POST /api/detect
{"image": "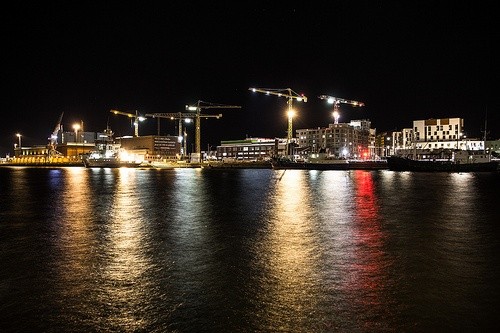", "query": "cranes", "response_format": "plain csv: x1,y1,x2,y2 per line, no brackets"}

49,112,65,150
109,109,147,137
185,100,242,153
248,87,308,141
145,111,219,152
319,95,365,124
